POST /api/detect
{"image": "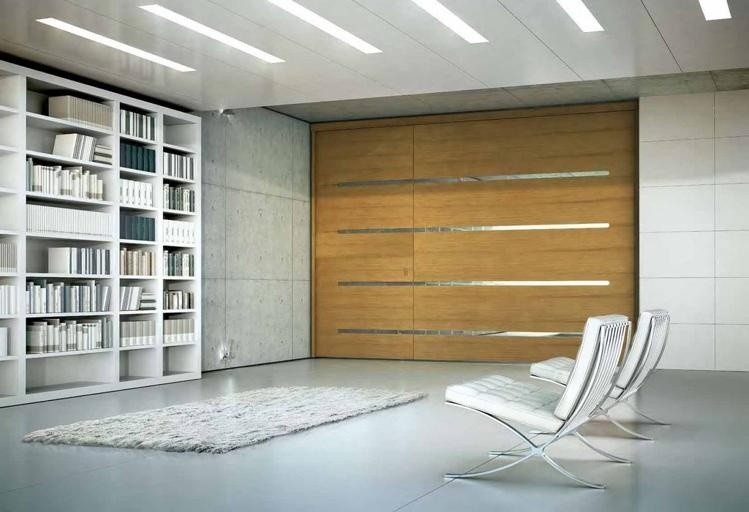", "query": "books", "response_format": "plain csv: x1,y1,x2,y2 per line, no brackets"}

1,82,198,355
